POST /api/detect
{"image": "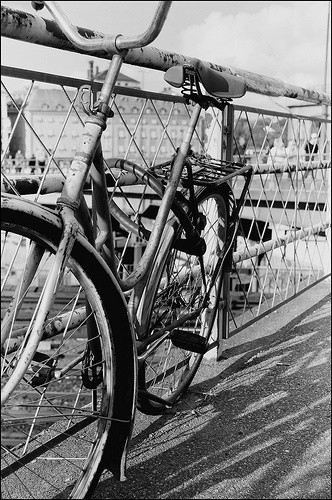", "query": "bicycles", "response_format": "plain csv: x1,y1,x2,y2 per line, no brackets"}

0,1,253,500
